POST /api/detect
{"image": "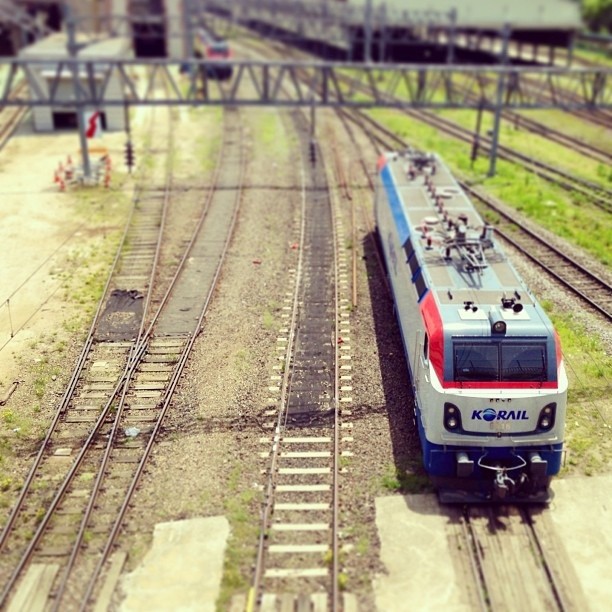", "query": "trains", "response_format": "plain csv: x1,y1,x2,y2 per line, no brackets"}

192,25,233,78
375,150,568,503
